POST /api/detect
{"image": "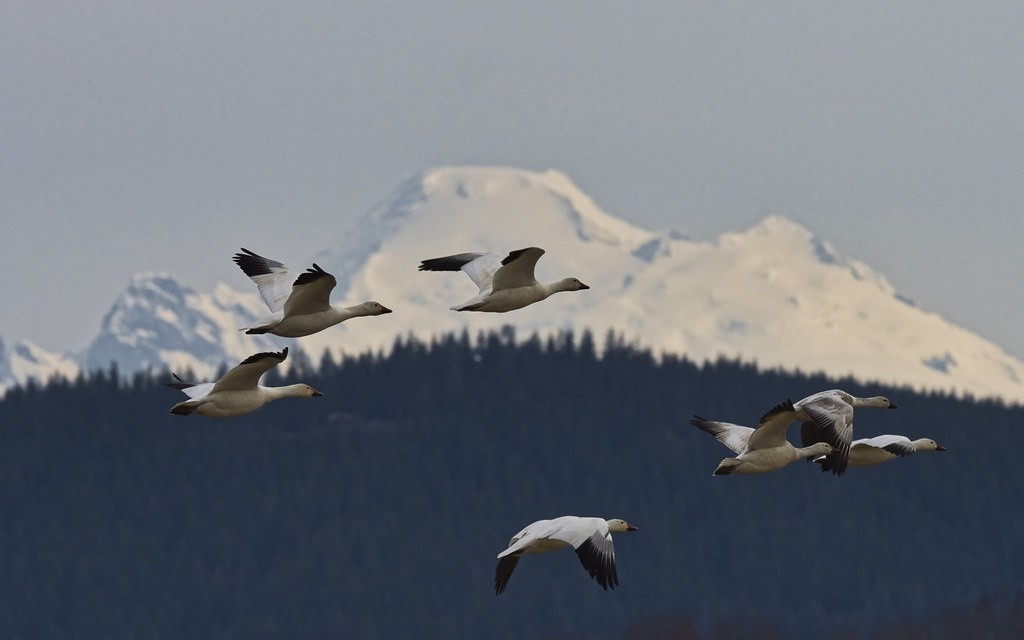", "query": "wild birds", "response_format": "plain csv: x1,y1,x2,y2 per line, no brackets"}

688,398,842,477
231,248,393,338
418,247,590,313
163,347,325,419
811,434,946,467
793,389,899,477
494,515,639,596
